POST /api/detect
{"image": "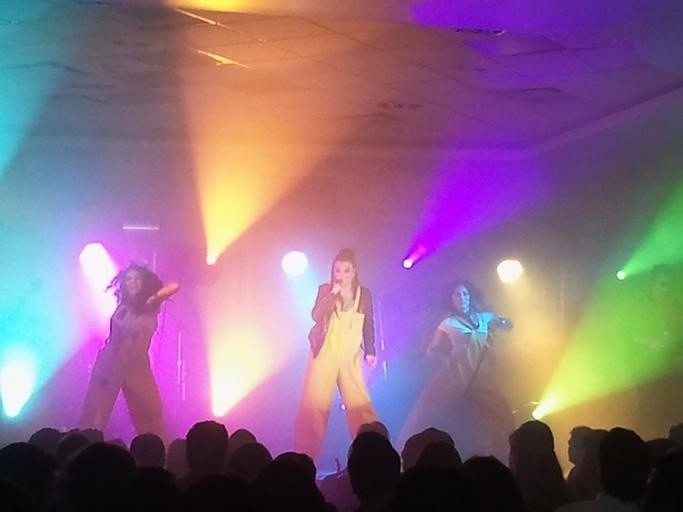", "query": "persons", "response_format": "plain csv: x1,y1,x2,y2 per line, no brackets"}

78,260,180,452
0,418,683,512
293,248,376,466
394,281,514,456
